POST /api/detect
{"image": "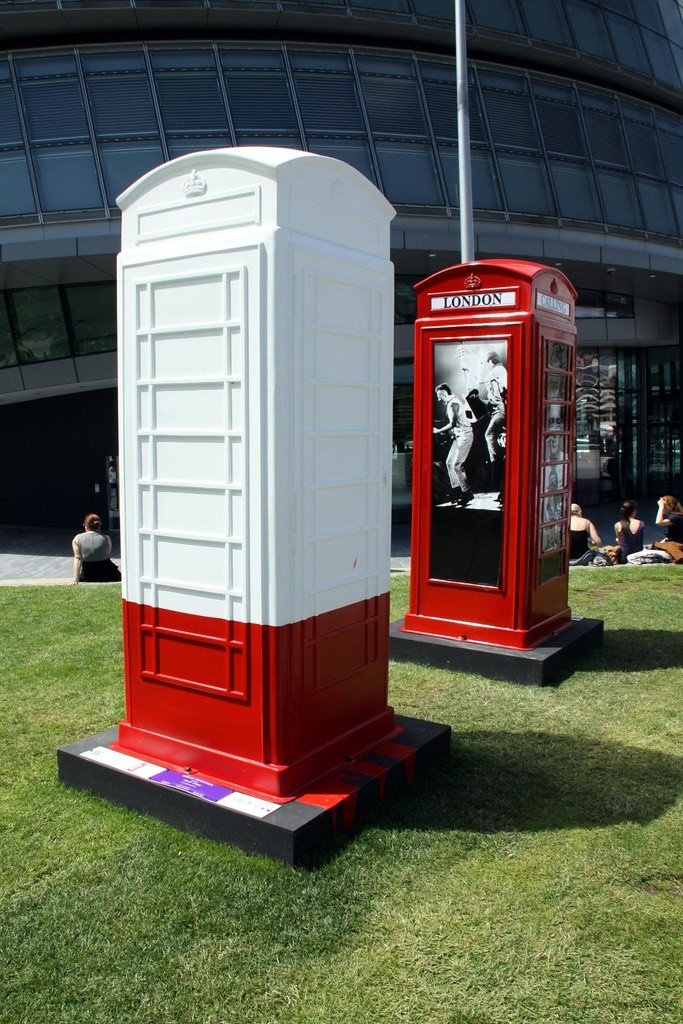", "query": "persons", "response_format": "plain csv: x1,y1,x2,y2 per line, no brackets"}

570,503,601,565
433,384,474,504
71,512,123,584
544,387,566,551
614,499,645,564
655,496,683,554
476,350,506,490
